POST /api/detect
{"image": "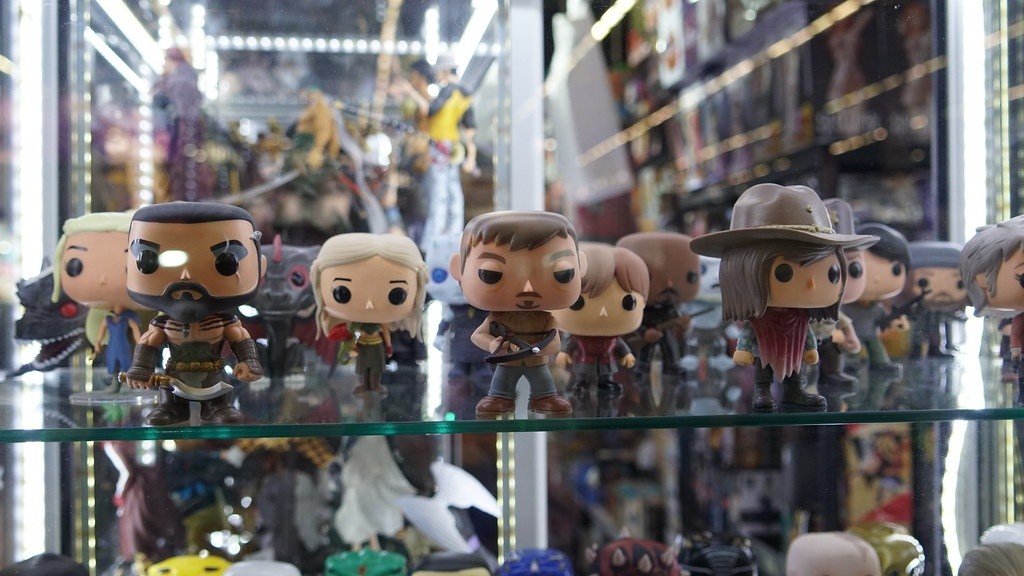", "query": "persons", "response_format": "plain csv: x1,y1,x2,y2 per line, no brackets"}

840,219,913,379
309,232,430,395
943,215,1024,400
50,207,155,394
691,182,872,417
553,238,653,414
117,201,267,425
449,211,590,415
613,230,701,379
409,52,477,252
819,197,864,393
903,236,969,360
